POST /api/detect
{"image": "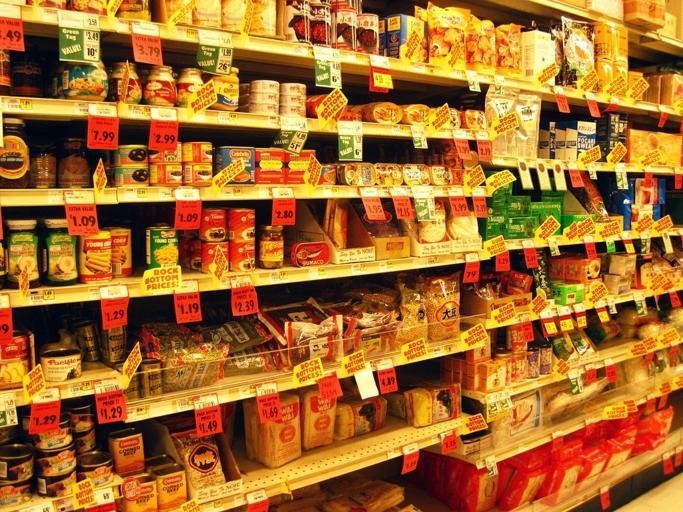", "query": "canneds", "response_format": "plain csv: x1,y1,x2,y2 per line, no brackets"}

1,208,284,289
1,315,162,402
1,404,187,512
2,118,307,188
1,47,306,120
